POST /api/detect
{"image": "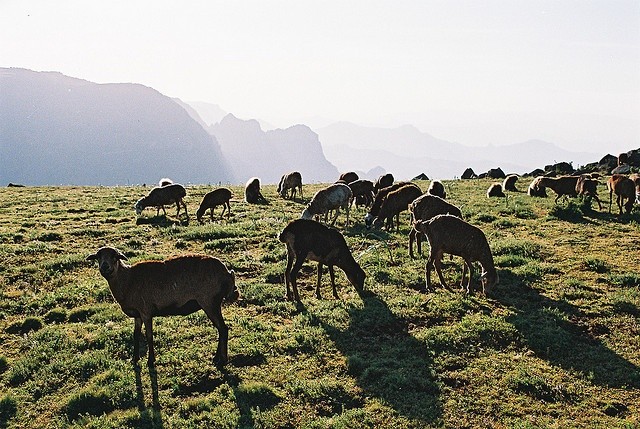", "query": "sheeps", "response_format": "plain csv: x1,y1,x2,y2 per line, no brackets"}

280,171,304,202
86,246,240,367
537,175,587,205
134,183,189,219
372,173,394,196
300,183,353,226
245,179,266,203
355,193,374,207
574,176,602,210
374,184,424,232
276,175,293,195
364,182,421,229
339,171,358,185
629,172,640,203
607,174,636,214
278,219,366,303
348,179,374,208
407,194,464,260
196,187,234,222
328,179,348,218
413,212,500,296
